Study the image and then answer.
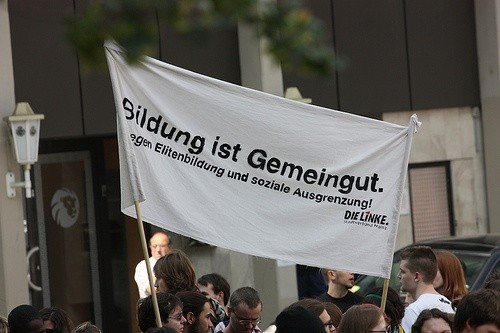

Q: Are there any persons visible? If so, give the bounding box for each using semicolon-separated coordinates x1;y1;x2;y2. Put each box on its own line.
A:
396;246;500;333
276;267;404;333
134;231;230;333
216;287;262;333
0;305;101;333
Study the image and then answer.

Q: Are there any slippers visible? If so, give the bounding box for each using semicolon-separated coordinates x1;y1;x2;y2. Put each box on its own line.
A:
167;313;183;321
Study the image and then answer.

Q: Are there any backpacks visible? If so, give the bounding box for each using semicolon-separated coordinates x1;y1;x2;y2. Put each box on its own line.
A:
204;296;230;333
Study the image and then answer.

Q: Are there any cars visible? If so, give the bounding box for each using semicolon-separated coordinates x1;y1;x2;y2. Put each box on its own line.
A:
348;233;500;298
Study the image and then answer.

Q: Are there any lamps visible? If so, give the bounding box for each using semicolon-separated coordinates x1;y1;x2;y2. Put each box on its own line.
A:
285;87;312;104
3;102;44;199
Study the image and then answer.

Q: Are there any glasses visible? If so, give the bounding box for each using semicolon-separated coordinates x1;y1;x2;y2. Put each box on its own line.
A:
322;319;334;329
230;307;262;325
